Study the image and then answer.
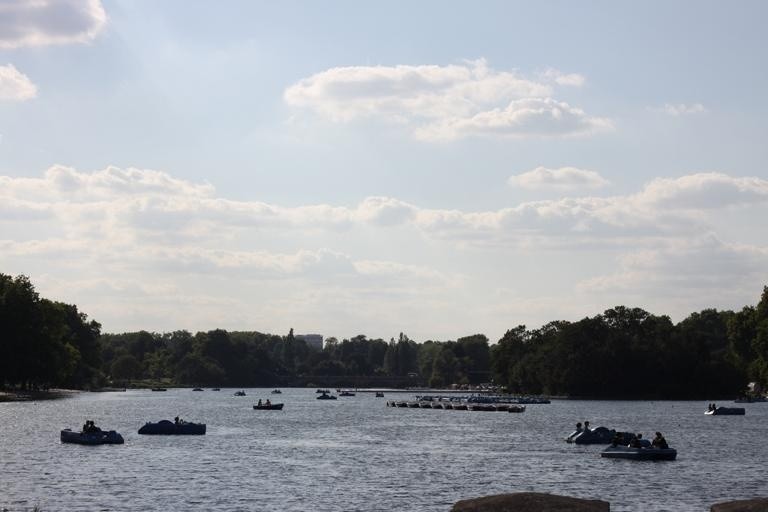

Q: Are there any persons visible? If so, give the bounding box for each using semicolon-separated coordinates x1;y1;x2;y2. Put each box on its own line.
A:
258;398;262;406
265;398;270;406
83;419;89;431
575;420;668;449
89;421;96;432
174;416;178;424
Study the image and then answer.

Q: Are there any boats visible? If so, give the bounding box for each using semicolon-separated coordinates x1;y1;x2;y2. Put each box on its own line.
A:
704;406;745;415
138;420;206;435
735;397;768;403
316;391;355;399
61;428;124;444
376;394;384;397
565;427;677;460
253;403;284;410
151;389;282;396
387;396;550;412
